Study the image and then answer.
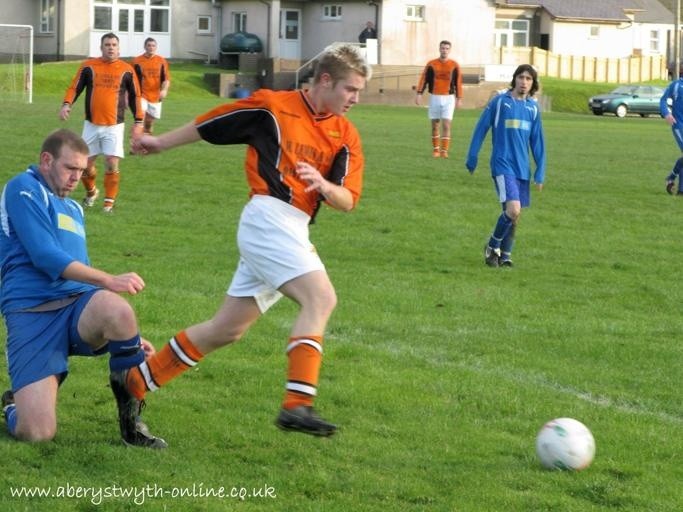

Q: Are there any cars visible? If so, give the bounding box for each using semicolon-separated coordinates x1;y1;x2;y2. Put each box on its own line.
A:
588;85;671;118
667;58;683;81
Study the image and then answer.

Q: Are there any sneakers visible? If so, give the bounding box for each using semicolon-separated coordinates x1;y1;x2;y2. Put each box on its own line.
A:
432;151;449;159
121;416;169;450
1;390;15;423
81;187;99;209
484;245;513;268
103;198;114;214
110;367;146;446
273;404;337;438
665;177;683;197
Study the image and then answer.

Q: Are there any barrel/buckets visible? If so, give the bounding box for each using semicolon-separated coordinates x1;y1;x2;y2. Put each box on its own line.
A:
235;89;250;99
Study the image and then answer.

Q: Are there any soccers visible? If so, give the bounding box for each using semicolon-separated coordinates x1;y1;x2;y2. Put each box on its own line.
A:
536;418;595;472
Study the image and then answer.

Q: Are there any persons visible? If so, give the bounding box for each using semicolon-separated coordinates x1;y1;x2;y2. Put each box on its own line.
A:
0;127;170;449
55;32;144;215
108;40;370;441
460;62;548;271
659;77;682;198
357;21;376;48
413;39;464;160
127;38;170;157
